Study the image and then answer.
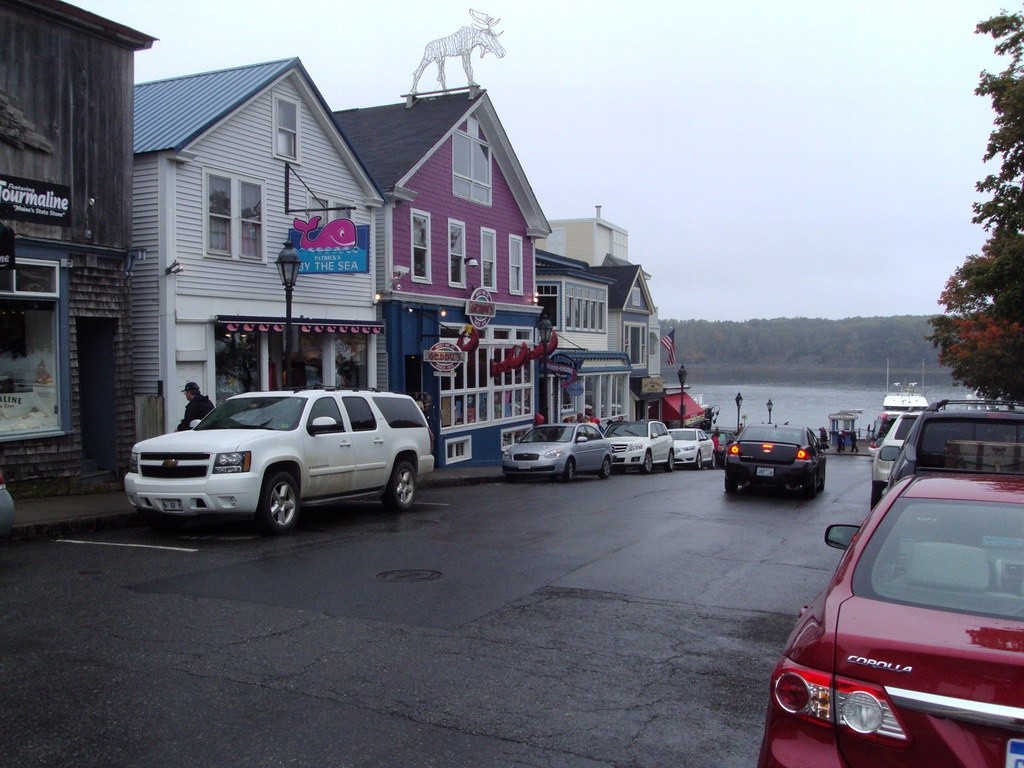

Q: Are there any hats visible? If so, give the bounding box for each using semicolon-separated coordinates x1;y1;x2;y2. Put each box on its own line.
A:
181;382;199;392
839;432;843;434
713;431;720;436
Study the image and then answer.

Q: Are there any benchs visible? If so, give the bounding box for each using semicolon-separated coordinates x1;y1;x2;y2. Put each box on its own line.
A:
896;543;1024;601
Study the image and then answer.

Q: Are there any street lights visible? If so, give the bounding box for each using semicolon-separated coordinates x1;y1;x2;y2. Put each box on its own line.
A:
275;244;303;390
678;364;687;429
735;391;742;436
766;398;774;422
537;314;553;424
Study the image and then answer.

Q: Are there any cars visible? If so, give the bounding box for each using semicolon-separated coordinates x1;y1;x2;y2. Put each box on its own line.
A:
502;423;614;480
705;430;737;470
725;425;822;496
756;470;1023;768
669;428;716;469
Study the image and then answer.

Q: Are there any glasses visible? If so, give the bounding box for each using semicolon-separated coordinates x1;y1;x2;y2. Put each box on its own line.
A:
183;393;188;395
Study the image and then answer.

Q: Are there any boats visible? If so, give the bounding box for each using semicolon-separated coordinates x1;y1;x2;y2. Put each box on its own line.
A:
884;357;928;409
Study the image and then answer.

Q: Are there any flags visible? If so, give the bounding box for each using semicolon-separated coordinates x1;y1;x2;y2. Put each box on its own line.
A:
660;327;676;369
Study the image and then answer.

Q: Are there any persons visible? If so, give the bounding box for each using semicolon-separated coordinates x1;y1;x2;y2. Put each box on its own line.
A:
607;416;624;426
174;381;215;432
710;430;720;453
739;422;744;434
818;420;876;453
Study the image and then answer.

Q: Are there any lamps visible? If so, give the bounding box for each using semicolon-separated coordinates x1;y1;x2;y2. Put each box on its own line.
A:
405;305;413;313
389;265;410;282
464;258;478;269
87;197;99;211
166;259;184;275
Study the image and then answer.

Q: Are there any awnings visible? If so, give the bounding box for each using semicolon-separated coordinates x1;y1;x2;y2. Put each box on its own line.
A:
663;392;705;425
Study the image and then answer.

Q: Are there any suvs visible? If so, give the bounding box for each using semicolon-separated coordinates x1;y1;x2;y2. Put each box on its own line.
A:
886;402;1024;520
870;410;919;511
602;419;674;473
868;411;905;458
124;389;432;534
